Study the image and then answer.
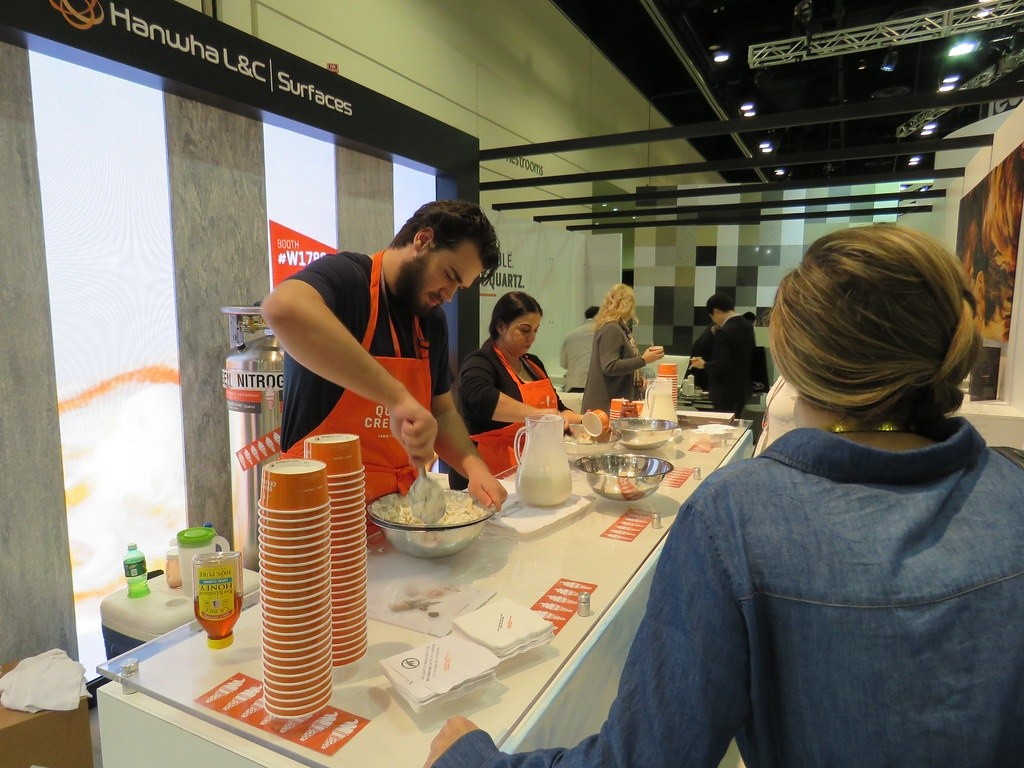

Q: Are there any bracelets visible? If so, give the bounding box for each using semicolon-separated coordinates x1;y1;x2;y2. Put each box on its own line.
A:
581;418;583;424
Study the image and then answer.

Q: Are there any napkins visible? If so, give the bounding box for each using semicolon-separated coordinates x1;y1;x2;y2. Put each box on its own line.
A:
379;599;558;714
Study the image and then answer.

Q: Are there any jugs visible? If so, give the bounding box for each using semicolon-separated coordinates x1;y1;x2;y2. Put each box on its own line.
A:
175;527;231;599
640;377;679;425
513;413;573;507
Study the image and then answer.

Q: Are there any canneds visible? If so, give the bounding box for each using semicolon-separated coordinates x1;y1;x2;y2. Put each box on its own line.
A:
165;549;183;588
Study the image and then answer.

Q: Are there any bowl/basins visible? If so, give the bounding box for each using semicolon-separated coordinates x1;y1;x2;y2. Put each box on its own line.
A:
368;487;497;559
574;453;674;502
609;417;680;451
581;409;610;438
563;423;623;462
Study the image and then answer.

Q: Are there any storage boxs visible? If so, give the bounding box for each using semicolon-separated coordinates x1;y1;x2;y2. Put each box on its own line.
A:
0;660;94;768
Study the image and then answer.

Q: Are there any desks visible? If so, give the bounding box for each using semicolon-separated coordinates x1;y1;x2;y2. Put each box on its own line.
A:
676;387;769;410
97;418;756;768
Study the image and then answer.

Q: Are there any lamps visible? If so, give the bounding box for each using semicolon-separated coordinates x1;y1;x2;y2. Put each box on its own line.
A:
879;50;900;71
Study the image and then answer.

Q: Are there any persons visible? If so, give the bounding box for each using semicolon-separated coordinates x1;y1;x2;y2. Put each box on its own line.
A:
260;199;507;538
580;284;665;417
422;225;1024;768
449;291;612;490
761;376;843;451
691;295;758;418
561;306;599;393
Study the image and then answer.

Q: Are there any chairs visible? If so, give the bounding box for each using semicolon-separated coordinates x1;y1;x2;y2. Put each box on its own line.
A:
642;354;691;385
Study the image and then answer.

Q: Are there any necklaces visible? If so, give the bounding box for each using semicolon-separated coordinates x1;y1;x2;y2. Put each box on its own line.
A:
824;419;901;434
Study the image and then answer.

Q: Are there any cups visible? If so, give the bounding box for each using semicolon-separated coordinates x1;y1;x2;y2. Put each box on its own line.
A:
657;363;678;411
702;392;709;399
649;346;663;354
609;398;644;421
690;356;701;364
256;434;369;720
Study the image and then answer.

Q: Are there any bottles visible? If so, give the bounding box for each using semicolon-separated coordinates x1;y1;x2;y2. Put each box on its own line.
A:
166;537;182;588
620;404;638;418
191;551;244;650
682;374;695;396
122;543;151;599
